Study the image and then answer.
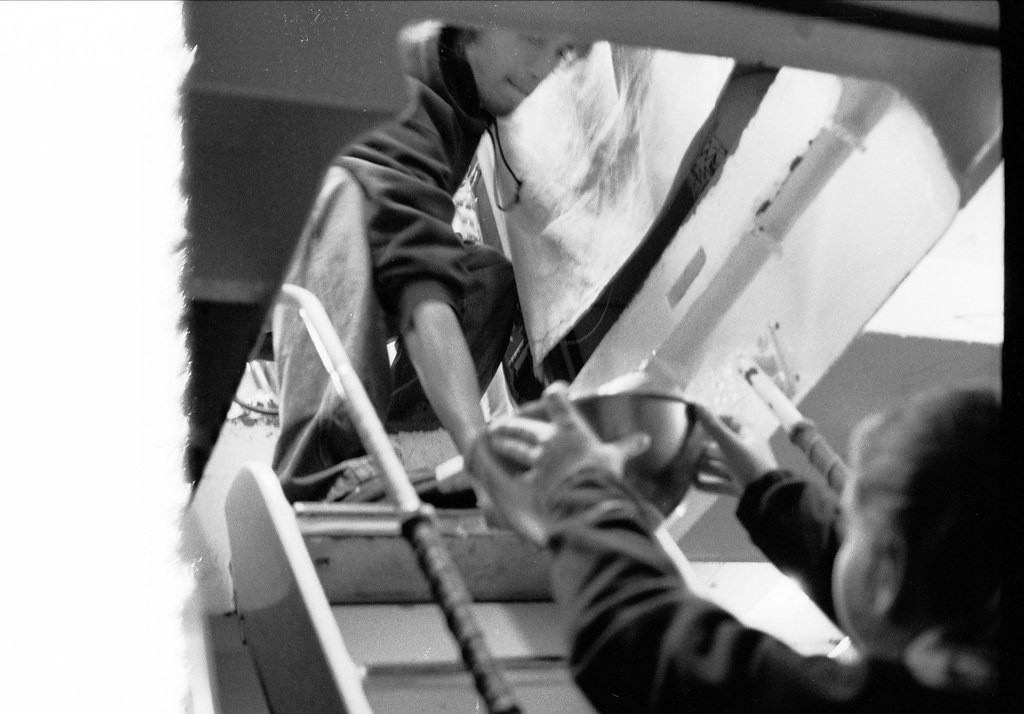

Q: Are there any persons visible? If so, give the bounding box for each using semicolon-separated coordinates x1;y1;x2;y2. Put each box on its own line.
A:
488;381;1024;714
319;20;575;531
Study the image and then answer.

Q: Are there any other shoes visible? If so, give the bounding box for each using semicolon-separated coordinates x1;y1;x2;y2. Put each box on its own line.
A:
324;455;477;510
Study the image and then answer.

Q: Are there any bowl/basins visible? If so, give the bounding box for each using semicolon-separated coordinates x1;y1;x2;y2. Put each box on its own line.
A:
491;391;710;529
